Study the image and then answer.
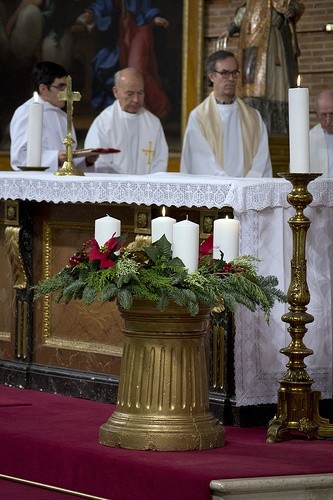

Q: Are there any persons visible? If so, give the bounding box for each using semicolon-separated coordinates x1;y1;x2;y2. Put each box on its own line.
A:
10;60;98;172
307;89;333;176
84;68;168;176
180;50;272;179
218;0;305;133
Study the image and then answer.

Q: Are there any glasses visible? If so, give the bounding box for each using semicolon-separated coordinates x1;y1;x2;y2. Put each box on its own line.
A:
48;84;67;91
213;69;240;79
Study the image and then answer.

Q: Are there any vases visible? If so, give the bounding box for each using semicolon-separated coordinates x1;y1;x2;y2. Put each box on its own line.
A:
99;295;227;451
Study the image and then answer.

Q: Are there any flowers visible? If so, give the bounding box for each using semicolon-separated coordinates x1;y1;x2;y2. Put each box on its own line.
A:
28;235;288;328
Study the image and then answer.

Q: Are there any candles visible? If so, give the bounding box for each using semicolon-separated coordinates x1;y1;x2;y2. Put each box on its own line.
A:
172;215;199;274
151;207;176;248
95;214;121;256
26;91;42;167
288;74;309;173
213;215;240;263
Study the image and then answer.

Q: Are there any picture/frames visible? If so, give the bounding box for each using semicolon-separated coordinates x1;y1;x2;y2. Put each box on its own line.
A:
0;0;204;171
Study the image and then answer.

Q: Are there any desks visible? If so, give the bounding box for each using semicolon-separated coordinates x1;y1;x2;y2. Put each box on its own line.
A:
0;170;333;428
